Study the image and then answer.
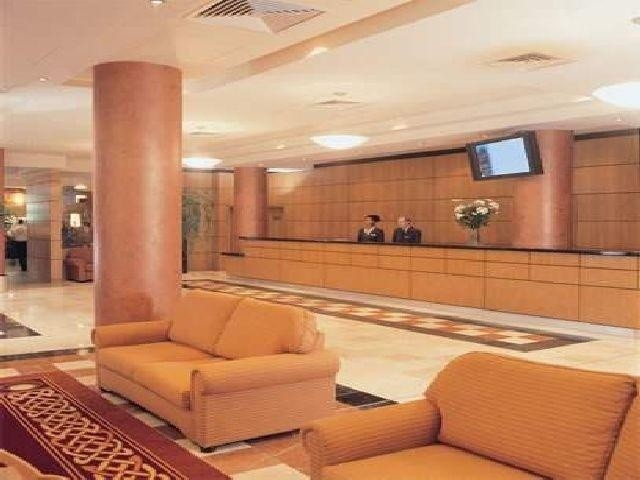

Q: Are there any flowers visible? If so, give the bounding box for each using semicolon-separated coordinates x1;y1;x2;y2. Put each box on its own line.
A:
450;197;500;231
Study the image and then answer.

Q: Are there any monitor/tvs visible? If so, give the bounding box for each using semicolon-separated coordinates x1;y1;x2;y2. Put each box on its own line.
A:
466;131;543;181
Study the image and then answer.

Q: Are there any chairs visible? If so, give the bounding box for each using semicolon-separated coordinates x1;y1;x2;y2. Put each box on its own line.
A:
65;246;93;281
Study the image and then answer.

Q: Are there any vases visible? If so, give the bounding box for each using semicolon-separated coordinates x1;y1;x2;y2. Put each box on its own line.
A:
466;228;479;244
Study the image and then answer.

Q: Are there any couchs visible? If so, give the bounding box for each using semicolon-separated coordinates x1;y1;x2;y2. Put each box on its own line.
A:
299;350;639;480
91;286;341;449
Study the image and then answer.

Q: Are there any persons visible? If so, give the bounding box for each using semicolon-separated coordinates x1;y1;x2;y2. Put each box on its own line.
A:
357;215;385;242
11;220;27;271
393;214;422;244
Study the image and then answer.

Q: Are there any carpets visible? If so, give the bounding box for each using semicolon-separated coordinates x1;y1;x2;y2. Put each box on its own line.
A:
1;369;236;480
